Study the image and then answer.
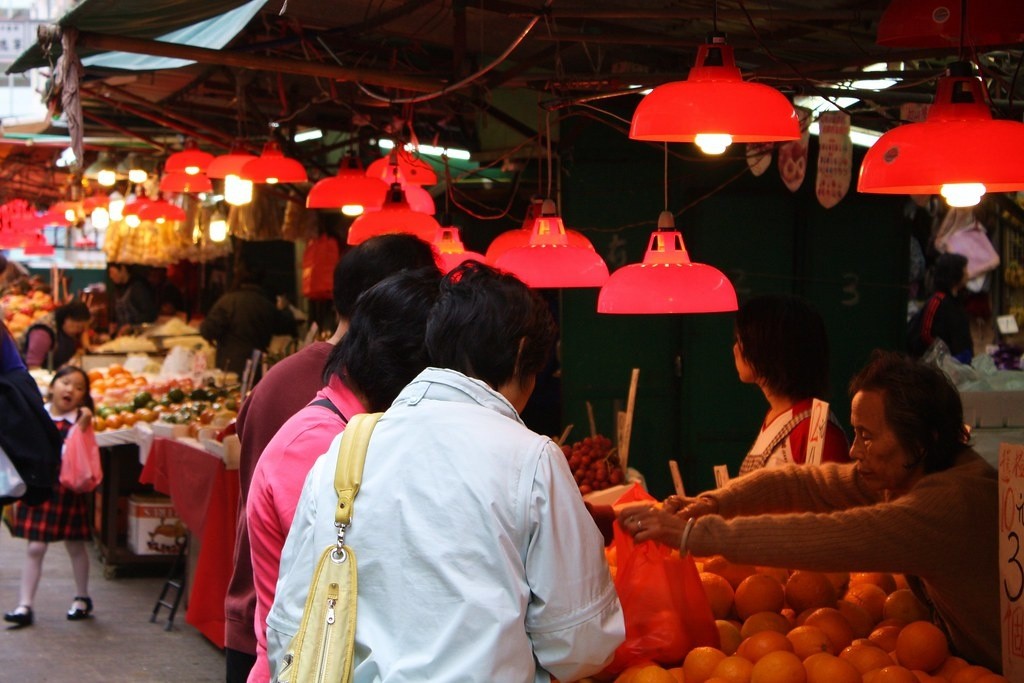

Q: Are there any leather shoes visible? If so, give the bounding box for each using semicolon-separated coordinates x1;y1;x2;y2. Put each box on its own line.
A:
4;605;32;624
68;597;92;619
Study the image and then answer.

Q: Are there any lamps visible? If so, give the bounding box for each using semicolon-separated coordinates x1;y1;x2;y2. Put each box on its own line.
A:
855;0;1024;208
594;143;740;316
628;0;802;158
0;87;611;289
874;0;1024;50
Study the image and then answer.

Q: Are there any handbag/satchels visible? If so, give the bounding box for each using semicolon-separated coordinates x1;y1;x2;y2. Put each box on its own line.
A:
942;222;1000;279
276;413;384;683
59;424;103;494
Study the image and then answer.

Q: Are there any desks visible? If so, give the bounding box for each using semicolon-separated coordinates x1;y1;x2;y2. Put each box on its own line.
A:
140;437;243;652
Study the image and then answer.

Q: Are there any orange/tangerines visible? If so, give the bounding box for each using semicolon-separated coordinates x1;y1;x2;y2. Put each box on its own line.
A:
550;546;1009;683
87;363;243;437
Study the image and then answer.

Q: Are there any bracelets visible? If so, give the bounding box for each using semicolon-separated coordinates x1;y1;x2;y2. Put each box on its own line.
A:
679;517;695;557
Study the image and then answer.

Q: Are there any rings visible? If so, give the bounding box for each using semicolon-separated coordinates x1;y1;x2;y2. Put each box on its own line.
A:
637;521;642;531
630;515;634;521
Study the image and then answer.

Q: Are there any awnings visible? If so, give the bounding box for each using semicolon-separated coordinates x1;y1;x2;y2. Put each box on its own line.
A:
383;153;537;198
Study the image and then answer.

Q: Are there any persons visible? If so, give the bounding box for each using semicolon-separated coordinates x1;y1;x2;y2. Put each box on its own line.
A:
196;234;627;683
17;303;91;372
0;254;97;626
108;264;159;323
621;293;1002;668
920;251;985;365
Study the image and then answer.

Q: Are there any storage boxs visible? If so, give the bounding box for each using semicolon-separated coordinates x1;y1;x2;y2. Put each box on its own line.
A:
960;370;1024;429
125;494;188;557
